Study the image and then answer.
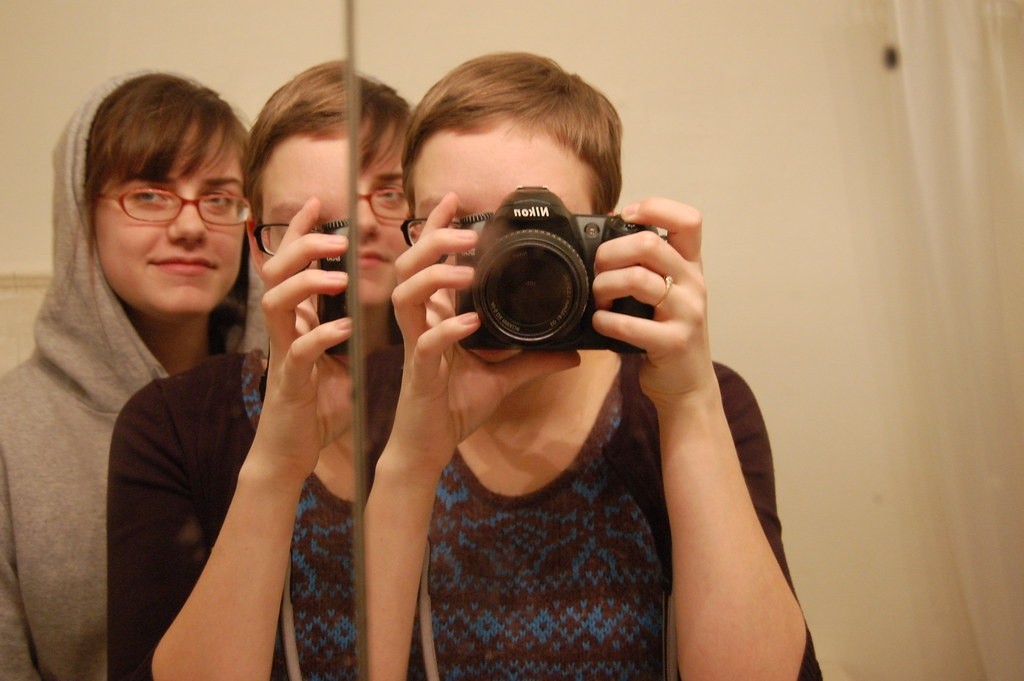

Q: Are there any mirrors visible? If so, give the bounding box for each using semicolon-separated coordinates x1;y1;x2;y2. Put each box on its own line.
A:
0;0;1024;681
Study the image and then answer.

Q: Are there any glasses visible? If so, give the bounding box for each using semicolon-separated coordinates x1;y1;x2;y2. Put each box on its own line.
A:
254;221;289;256
355;185;411;220
401;212;460;247
94;188;252;225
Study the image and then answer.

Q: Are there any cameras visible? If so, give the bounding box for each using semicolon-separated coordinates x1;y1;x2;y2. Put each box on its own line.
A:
316;251;349;357
456;185;661;353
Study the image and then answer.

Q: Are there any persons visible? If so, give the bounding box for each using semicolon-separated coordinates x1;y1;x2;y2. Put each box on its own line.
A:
1;63;412;681
361;53;829;681
